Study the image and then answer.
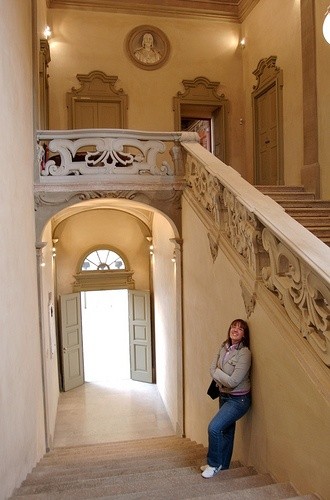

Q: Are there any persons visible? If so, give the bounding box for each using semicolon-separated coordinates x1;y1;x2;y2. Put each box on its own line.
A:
134;32;161;64
199;318;252;478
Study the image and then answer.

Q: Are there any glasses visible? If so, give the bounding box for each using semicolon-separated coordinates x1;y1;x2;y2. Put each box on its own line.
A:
231;325;243;331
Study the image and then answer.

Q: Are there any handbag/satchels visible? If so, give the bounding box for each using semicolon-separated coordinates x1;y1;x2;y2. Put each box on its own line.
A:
207;379;220;400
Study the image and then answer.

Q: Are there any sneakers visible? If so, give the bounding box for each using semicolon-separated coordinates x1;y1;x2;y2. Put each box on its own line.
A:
201;465;222;478
200;465;209;471
